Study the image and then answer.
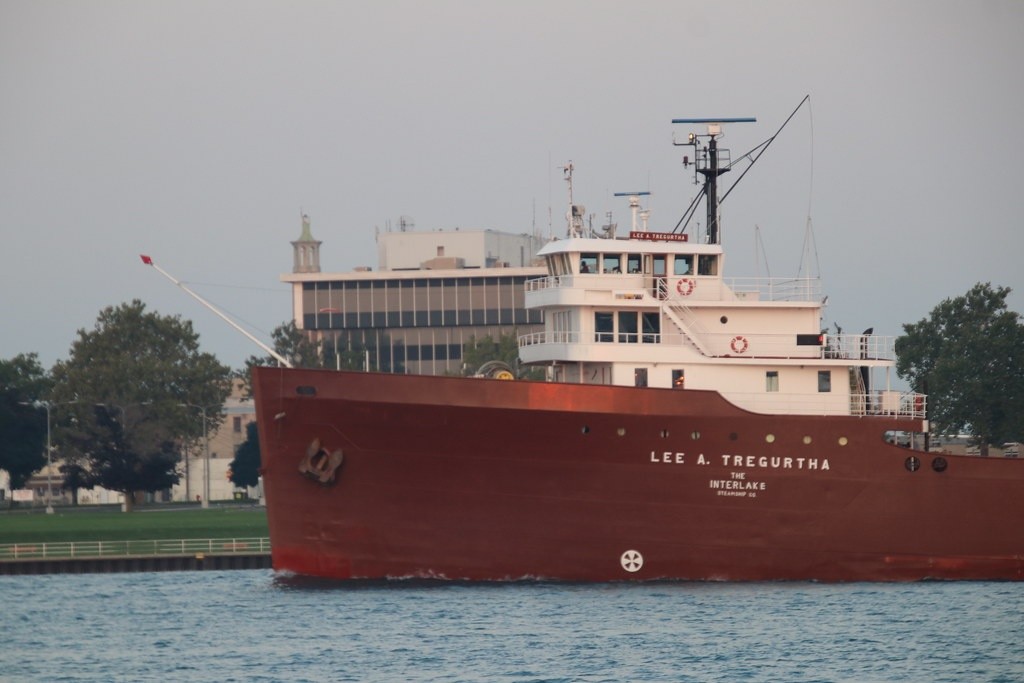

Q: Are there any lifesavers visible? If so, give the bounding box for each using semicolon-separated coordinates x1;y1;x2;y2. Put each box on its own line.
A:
730;335;749;353
676;278;694;295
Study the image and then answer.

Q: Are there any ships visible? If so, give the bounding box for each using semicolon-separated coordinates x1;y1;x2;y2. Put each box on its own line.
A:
140;94;1023;588
